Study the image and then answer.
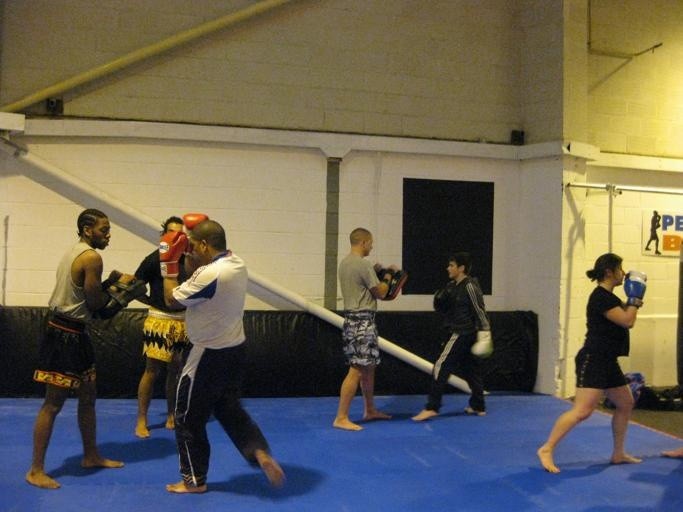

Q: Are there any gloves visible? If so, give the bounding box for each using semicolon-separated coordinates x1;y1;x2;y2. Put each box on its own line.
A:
158;213;210;278
471;330;494;360
624;270;648;306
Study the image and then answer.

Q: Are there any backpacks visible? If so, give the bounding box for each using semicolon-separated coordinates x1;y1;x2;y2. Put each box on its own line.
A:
604;372;660;411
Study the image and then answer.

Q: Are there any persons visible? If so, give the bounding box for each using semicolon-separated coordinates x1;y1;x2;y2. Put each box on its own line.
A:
129;215;189;438
22;208;147;490
535;253;647;475
411;251;494;423
158;211;286;495
332;228;409;432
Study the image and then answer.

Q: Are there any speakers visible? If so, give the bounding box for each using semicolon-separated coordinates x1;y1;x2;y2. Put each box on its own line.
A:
46;97;63;115
510;129;525;145
